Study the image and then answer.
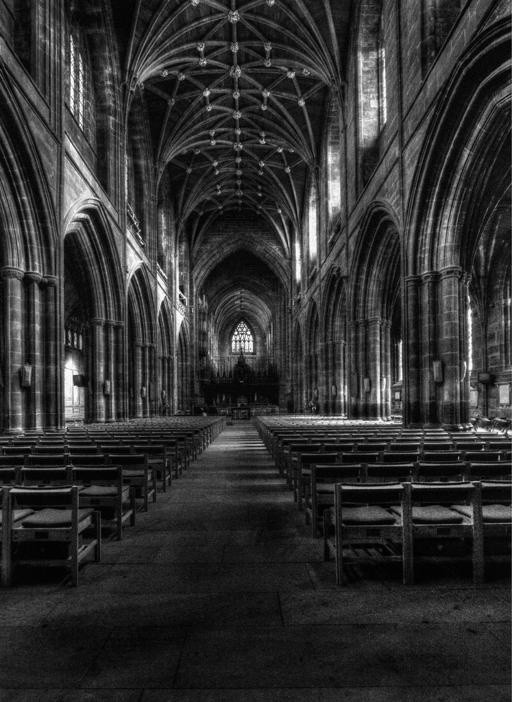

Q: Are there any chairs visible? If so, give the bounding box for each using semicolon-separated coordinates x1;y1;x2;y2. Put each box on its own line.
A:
255;415;510;589
0;415;227;590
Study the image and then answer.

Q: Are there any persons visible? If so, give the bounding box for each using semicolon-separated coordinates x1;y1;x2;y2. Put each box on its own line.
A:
287;395;293;413
